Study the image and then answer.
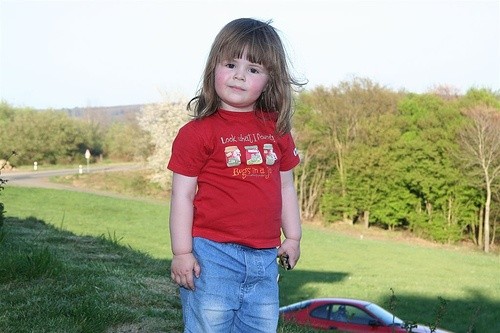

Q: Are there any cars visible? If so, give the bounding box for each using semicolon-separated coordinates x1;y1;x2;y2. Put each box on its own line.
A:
279;297;455;333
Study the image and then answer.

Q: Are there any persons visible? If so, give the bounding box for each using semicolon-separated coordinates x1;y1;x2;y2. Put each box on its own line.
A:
166;18;310;332
